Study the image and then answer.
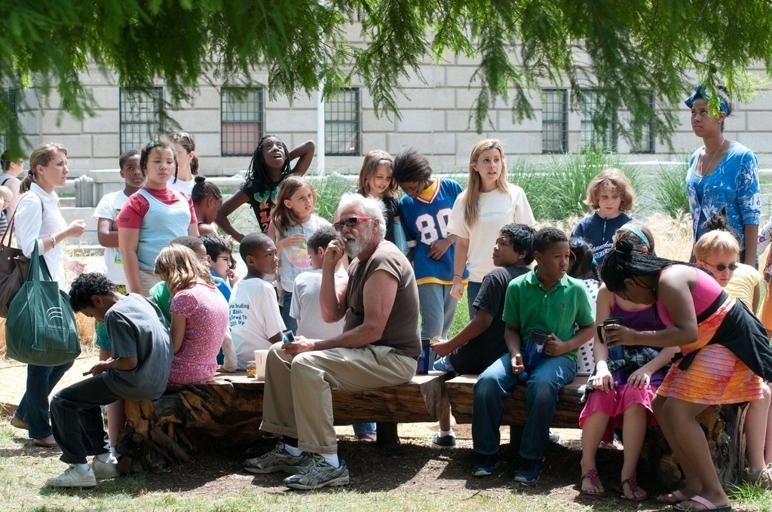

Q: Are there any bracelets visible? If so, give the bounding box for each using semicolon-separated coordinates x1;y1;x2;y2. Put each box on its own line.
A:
447;236;455;244
453;273;463;280
49;235;56;248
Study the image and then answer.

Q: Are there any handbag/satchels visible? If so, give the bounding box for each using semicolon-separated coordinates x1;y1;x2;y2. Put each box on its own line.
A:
5;280;82;367
0;244;31;318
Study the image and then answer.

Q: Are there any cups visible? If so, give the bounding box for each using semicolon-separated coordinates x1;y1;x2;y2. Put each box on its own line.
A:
415;336;437;373
521;328;548;369
596;318;627;361
254;350;269;378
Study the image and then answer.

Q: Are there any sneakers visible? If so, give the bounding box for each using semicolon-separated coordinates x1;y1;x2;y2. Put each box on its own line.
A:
514;455;545;482
472;464;495;477
433;433;455;446
243;441;311;474
91;456;120;479
32;434;57;447
46;465;97;487
11;417;29;429
357;434;376;441
282;453;350;490
743;463;772;490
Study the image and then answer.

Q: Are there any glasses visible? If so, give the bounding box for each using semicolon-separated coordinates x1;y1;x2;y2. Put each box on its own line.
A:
334;215;375;232
216;255;231;264
702;260;738;271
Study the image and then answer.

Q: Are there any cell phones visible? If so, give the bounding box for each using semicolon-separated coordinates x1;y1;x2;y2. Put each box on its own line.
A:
283;330;296;342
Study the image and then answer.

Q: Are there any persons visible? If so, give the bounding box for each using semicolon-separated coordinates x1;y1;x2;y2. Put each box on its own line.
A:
167;132;200;198
392;147;469;375
1;148;24;235
428;221;538;447
108;242;239;459
600;230;772;512
578;223;681;501
288;225;350;342
191;175;224;237
199;233;239;303
44;272;175;488
227;232;288;373
701;214;762;320
241;192;422;491
569;167;636;267
267;176;333;336
8;142;88;448
685;82;760;274
353;148;400;443
92;148;147;296
691;228;771;491
469;226;595;484
214;134;316;244
115;140;200;298
0;186;16;245
756;216;771;346
148;235;211;325
446;138;536;321
566;238;603;376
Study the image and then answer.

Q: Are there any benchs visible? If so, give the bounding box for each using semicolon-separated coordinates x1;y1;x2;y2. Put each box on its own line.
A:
444;364;751;483
115;371;446;477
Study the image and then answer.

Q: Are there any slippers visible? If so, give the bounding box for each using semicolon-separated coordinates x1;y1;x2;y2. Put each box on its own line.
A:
577;469;608;495
673;495;732;512
656;489;688;504
614;478;649;502
